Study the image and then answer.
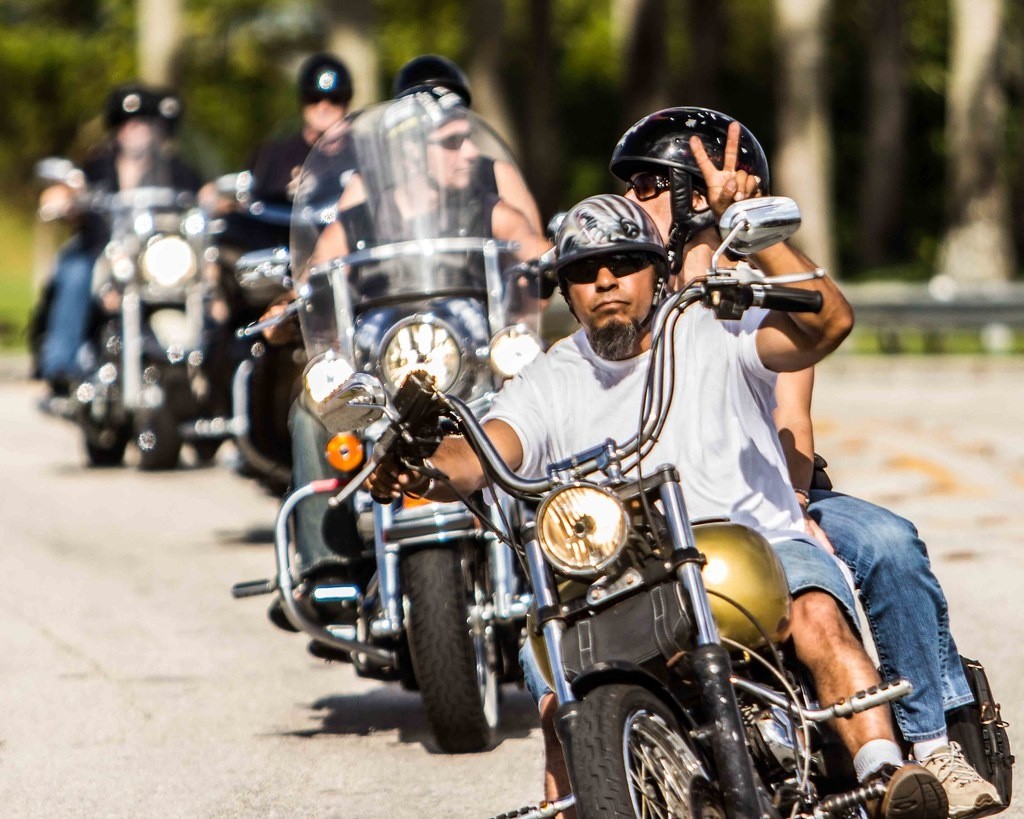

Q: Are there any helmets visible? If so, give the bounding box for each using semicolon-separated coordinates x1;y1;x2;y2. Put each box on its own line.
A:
553;194;669;306
395;54;470;107
378;84;463;146
297;54;351;101
609;106;770;199
108;88;181;128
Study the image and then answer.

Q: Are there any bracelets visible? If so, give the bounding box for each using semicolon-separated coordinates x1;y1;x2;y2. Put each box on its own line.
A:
793;488;808;499
402;458;435;500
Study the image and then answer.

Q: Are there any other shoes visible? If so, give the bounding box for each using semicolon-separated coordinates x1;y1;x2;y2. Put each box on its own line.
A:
268;573;374;632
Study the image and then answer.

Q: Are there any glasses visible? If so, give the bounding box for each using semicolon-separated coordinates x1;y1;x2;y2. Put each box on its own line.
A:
562;250;660;284
624;172;707;201
413;128;472;150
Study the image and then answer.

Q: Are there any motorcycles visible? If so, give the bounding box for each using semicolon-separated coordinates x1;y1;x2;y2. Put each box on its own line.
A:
197;108;393;490
229;82;559;757
315;198;1017;819
40;105;233;471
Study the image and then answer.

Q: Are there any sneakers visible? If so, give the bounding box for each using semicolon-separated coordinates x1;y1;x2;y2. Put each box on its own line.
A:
924;740;1004;817
862;762;950;819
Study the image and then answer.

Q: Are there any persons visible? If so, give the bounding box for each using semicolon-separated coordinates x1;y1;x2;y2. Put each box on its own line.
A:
38;84;237;411
336;52;549;331
259;88;558;630
610;106;1005;819
199;56;357;419
360;121;950;819
